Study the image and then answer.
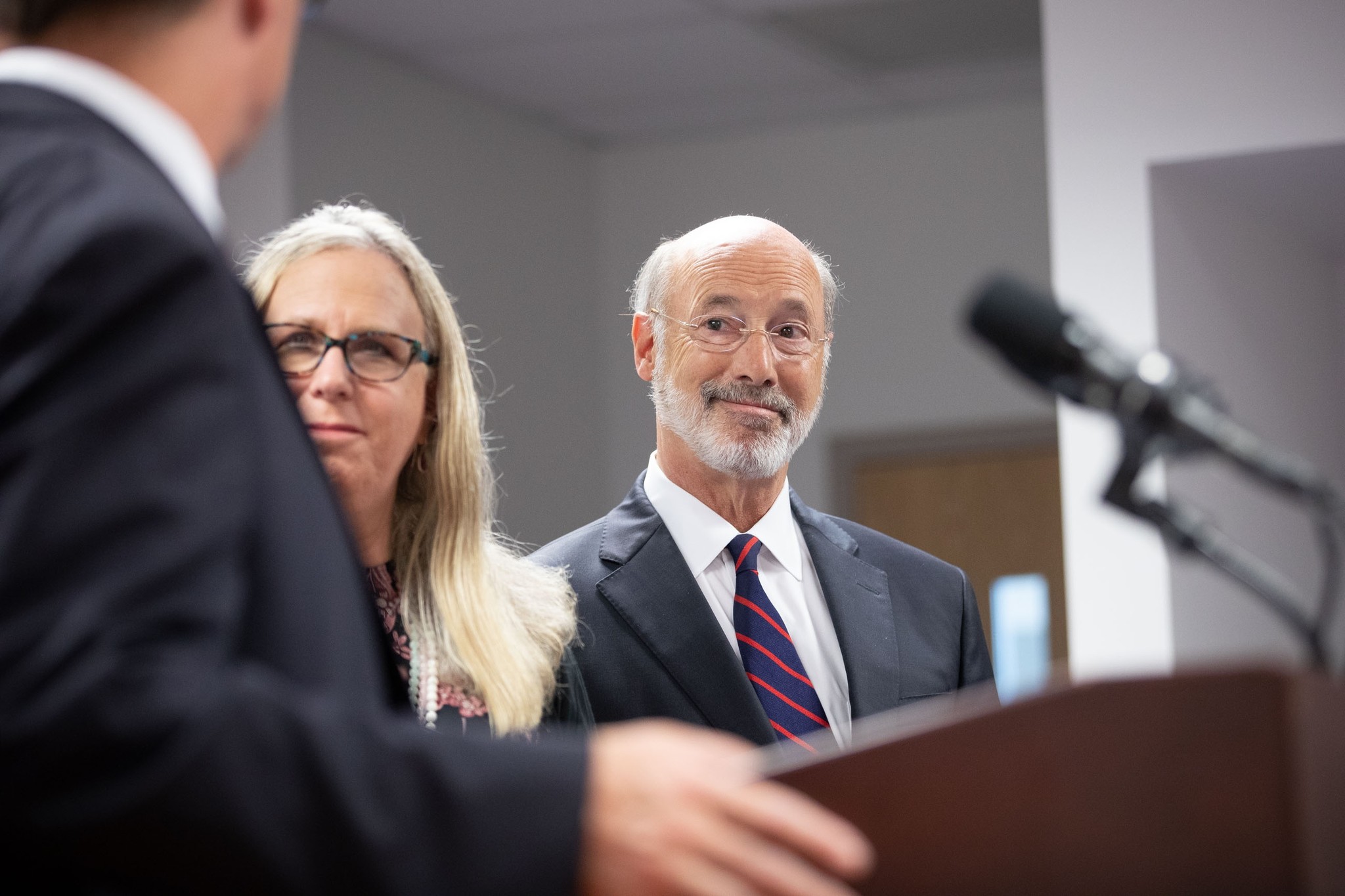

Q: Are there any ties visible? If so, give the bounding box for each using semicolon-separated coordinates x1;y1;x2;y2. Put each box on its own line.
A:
724;534;840;759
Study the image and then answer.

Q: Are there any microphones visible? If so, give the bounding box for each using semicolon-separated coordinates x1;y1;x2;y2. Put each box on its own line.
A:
966;276;1326;505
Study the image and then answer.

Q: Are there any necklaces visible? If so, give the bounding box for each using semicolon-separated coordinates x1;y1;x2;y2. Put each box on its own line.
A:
406;562;439;730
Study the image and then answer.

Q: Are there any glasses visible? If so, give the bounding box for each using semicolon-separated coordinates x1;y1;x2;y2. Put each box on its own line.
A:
647;307;830;360
264;322;439;382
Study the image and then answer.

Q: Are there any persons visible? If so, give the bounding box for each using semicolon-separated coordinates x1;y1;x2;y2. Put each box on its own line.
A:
518;214;1000;751
0;0;873;896
239;199;597;742
